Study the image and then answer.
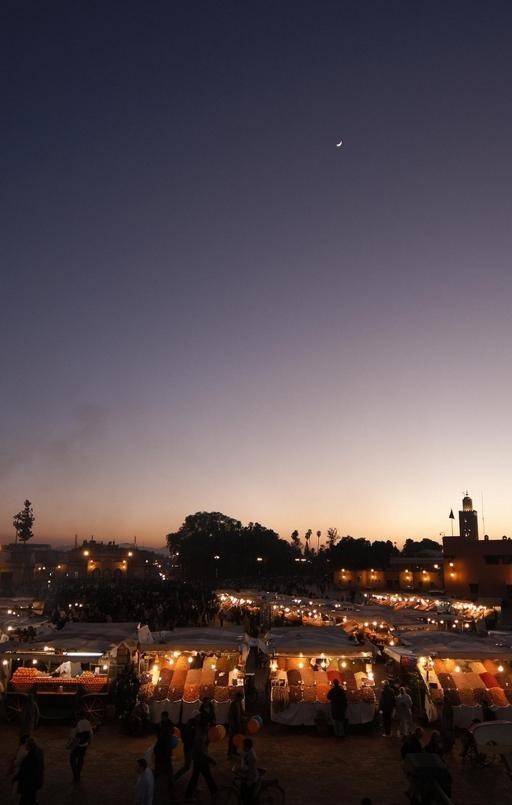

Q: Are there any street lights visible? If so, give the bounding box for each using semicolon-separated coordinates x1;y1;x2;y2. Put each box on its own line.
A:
214;555;220;579
256;557;263;592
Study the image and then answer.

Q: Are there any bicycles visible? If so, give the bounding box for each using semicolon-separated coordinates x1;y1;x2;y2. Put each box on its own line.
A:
217;777;286;805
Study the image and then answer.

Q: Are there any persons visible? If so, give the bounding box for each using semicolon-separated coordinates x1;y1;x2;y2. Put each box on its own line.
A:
109;680;286;805
327;680;485;805
12;694;93;805
